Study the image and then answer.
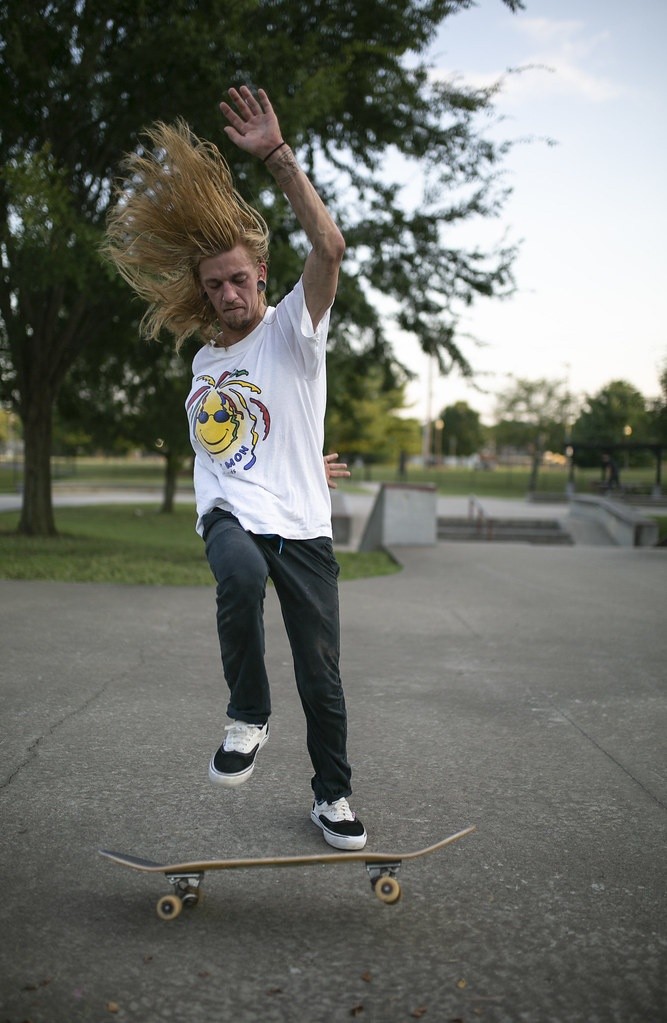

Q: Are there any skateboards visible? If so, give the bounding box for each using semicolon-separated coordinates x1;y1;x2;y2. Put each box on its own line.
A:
93;822;482;924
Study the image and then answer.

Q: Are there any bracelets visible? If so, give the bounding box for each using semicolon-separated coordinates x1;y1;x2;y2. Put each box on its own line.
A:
263;142;285;164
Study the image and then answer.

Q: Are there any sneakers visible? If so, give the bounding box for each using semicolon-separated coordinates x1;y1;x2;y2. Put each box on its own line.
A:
208;719;270;787
311;796;368;850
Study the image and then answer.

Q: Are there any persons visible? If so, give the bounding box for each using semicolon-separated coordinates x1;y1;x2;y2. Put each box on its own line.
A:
600;451;609;480
607;458;620;487
104;84;368;849
